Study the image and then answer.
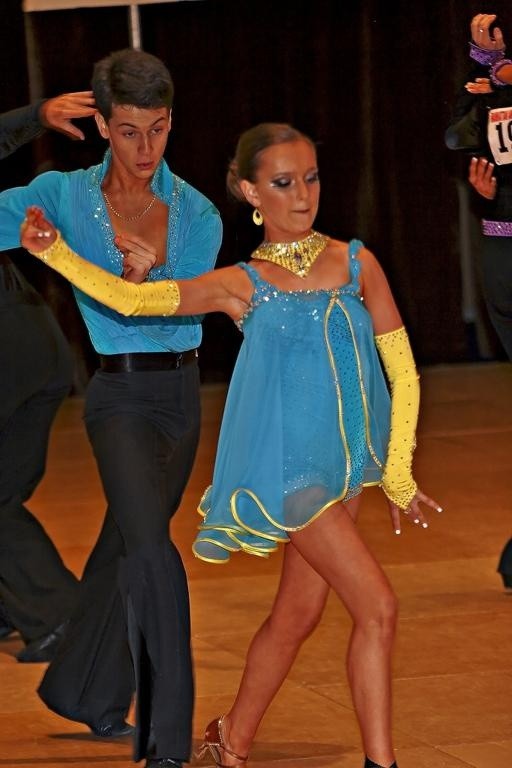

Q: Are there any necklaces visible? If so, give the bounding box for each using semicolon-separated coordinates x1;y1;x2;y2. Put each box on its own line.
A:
251;230;334;283
101;188;159;223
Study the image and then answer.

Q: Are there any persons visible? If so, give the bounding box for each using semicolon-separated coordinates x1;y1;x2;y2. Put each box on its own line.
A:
0;89;99;667
18;121;446;768
0;47;221;768
443;14;510;590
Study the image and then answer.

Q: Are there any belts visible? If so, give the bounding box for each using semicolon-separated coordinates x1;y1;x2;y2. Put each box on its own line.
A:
99;349;196;373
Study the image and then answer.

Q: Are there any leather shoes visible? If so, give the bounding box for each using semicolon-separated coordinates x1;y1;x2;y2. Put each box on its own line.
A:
145;759;183;768
87;722;135;737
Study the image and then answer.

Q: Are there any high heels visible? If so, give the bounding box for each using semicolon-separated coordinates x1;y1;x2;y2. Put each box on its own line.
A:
196;713;248;768
